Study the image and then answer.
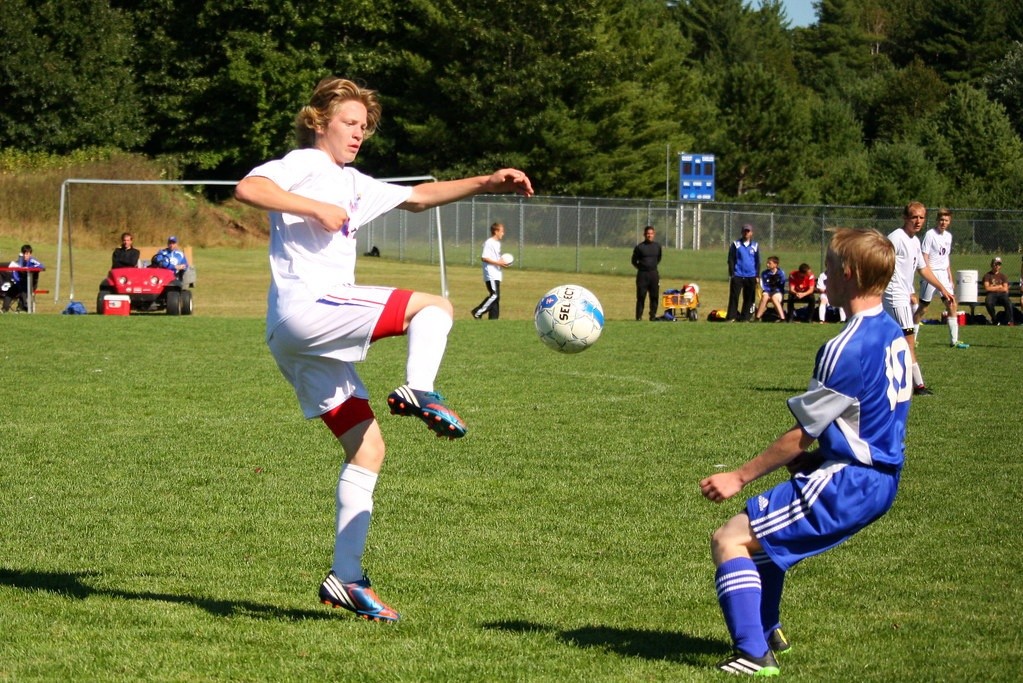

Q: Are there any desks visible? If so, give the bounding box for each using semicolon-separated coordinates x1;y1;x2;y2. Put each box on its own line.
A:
0;266;46;315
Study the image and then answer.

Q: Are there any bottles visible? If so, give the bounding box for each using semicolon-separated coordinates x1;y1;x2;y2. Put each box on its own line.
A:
18;252;22;268
143;260;147;269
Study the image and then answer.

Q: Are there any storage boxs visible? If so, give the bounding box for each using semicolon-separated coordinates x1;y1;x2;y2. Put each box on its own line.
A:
662;293;698;309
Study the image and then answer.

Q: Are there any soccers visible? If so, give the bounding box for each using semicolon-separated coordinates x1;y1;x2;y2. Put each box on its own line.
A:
533;285;606;354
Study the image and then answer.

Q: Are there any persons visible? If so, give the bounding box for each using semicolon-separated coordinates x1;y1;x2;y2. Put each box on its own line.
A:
753;256;786;323
471;222;509;320
982;256;1013;326
911;287;923;325
914;208;969;348
1019;264;1023;311
816;272;846;324
157;236;188;274
725;224;760;323
882;201;955;395
112;232;140;268
235;78;533;624
0;245;41;313
631;225;662;320
785;263;816;323
700;228;912;677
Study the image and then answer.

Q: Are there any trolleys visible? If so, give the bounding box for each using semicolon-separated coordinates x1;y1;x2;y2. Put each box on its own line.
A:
662;292;699;322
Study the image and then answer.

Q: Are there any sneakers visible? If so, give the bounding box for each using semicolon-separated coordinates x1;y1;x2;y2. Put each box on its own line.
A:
387;385;467;440
711;648;778;677
766;629;790;654
950;340;969;348
317;570;397;624
913;383;933;394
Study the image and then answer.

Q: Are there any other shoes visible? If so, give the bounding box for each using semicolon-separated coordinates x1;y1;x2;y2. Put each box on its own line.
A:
1008;321;1014;326
649;317;661;320
470;310;481;318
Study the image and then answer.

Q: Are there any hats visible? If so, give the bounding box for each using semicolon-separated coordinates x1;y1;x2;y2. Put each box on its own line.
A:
168;236;176;242
742;224;752;231
991;257;1002;264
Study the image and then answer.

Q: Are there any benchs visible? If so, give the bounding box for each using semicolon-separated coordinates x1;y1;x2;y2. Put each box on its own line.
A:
958;279;1023;319
766;277;820;314
